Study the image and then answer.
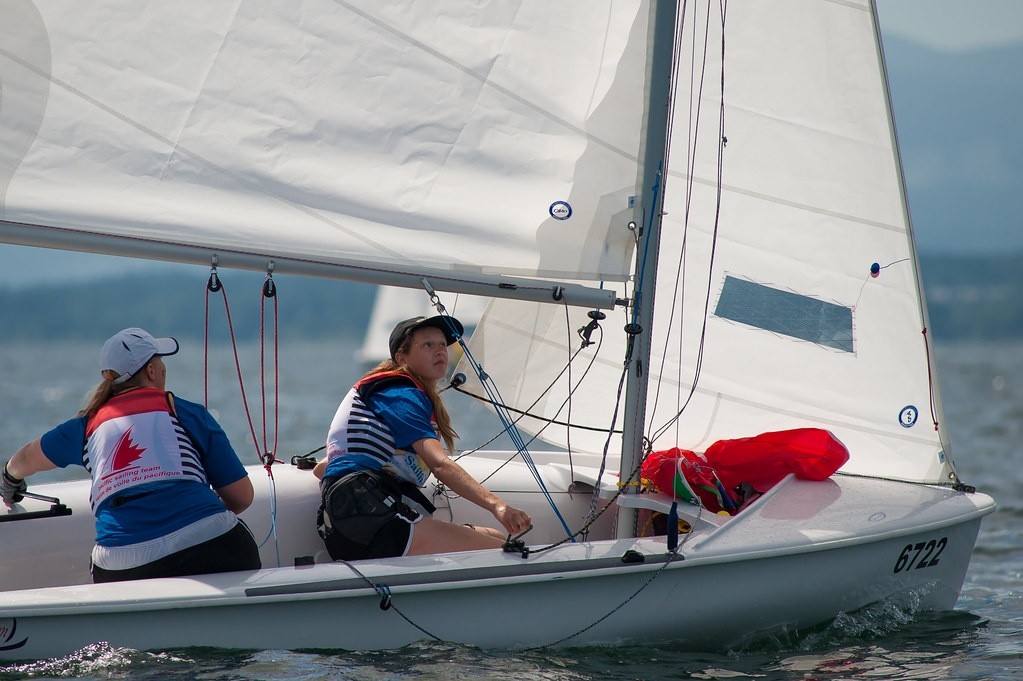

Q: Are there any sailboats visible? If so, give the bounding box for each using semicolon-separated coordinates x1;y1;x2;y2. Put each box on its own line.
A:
0;0;996;654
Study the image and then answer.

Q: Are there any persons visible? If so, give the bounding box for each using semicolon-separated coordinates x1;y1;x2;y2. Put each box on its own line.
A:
312;316;531;563
0;327;261;583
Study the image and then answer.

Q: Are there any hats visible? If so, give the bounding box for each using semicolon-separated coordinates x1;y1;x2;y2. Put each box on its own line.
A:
389;314;464;361
99;328;180;385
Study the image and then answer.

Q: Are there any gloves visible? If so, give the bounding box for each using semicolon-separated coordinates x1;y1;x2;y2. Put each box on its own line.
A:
0;461;28;505
